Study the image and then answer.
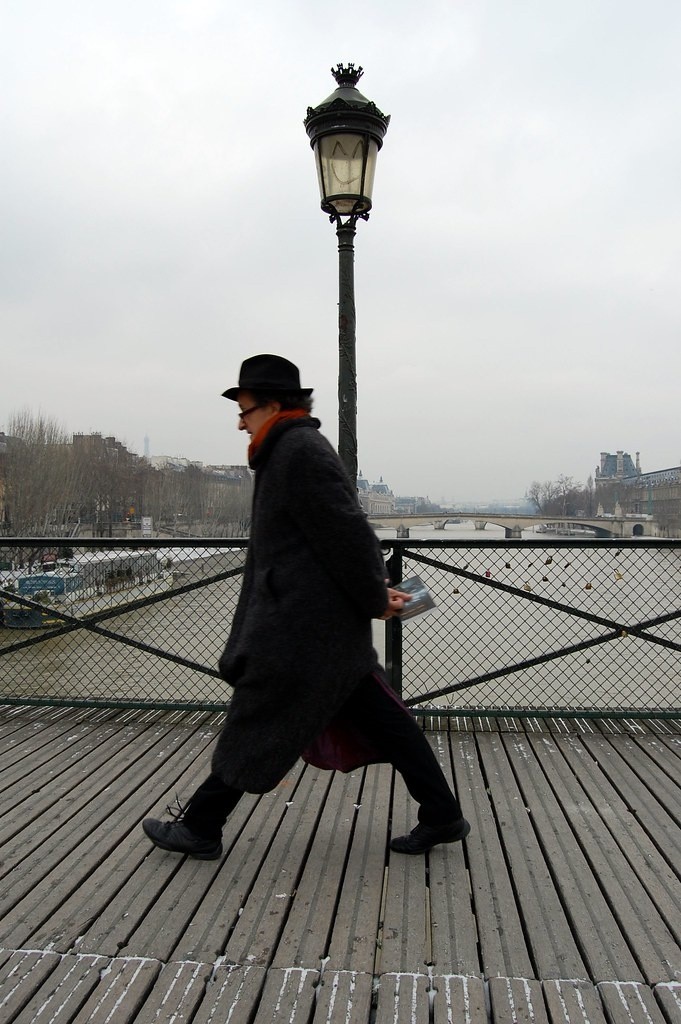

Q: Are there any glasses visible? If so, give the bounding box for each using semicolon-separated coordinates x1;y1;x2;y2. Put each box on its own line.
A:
238;399;272;419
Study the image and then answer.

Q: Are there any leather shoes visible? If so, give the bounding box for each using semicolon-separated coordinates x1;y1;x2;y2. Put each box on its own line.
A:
140;817;223;860
388;817;472;854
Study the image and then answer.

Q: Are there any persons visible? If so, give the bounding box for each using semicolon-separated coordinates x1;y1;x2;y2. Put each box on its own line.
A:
141;355;471;864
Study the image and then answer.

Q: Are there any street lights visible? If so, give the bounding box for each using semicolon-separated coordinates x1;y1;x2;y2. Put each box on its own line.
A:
305;59;395;506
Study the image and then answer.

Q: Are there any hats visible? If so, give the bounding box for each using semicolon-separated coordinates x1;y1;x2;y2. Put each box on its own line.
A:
222;354;313;402
